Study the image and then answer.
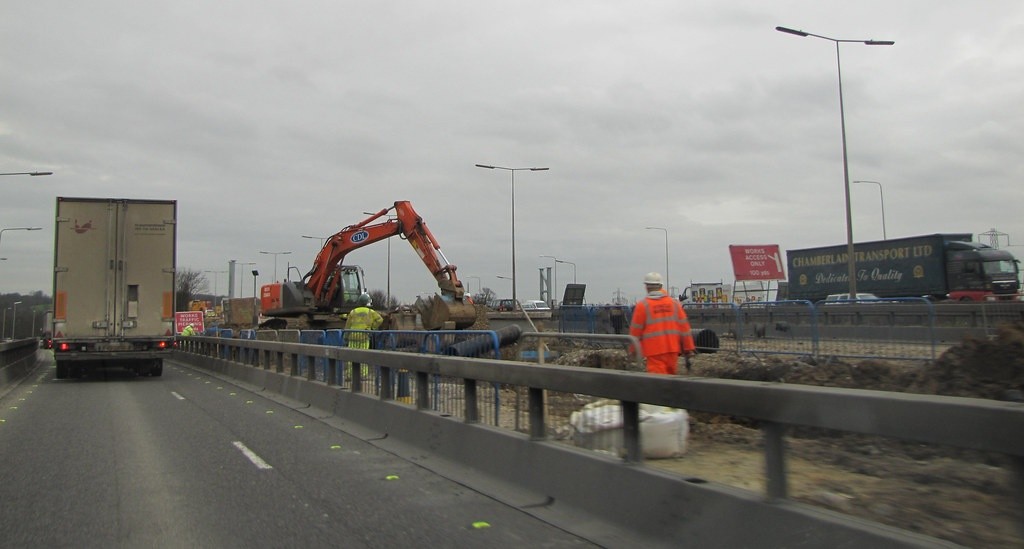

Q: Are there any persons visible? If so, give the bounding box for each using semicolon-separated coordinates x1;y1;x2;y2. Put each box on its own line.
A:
343;293;383;382
629;272;695;375
465;293;473;303
181;322;195;345
343;272;358;302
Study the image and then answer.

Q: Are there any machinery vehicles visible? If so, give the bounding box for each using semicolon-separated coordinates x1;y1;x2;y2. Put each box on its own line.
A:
256;198;477;365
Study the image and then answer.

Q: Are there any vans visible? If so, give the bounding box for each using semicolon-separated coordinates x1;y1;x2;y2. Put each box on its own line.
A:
825;292;881;304
517;300;553;311
490;298;515;311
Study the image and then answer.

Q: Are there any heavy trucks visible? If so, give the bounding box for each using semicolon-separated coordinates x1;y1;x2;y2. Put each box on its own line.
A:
786;232;1021;306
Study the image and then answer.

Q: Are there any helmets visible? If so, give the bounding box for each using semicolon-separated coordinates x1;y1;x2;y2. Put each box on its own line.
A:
358;293;371;307
190;323;194;328
644;272;663;285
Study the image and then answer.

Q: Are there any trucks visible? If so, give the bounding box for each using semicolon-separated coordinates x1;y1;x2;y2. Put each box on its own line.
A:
50;195;180;373
41;309;54;349
190;298;228;312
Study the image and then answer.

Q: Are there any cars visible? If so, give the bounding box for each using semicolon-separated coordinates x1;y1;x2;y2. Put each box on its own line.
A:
205;309;217;318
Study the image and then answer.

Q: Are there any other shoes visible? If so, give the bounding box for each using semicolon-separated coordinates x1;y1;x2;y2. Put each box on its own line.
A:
361;377;367;381
345;377;351;382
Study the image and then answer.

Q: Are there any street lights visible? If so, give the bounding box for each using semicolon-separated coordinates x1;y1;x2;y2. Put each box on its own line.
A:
644;225;671;296
775;25;897;303
302;235;326;250
2;308;13;342
539;254;562;311
466;276;482;293
233;261;257;297
362;211;397;317
203;270;228;308
555;259;576;284
473;163;549;313
259;251;292;282
12;301;23;342
